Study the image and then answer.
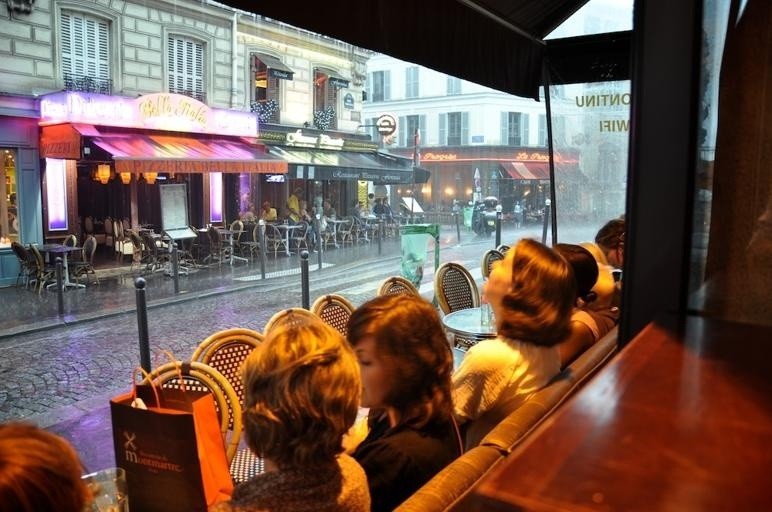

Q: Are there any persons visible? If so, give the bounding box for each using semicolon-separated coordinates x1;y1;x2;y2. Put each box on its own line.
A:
450;239;577;454
554;219;626;370
212;321;371;512
514;201;523;228
0;422;92;512
345;293;464;512
241;188;394;252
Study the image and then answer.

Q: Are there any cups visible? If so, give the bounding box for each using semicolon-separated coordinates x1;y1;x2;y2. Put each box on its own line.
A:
480;294;493;326
84;467;131;512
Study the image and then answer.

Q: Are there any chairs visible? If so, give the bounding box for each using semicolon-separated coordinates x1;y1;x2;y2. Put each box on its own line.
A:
9;200;432;294
145;245;622;493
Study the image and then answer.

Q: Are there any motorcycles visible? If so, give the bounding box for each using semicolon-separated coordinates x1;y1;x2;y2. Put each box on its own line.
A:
471;196;499;238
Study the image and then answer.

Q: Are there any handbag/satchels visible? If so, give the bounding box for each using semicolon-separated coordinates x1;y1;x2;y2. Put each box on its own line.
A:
109;349;236;511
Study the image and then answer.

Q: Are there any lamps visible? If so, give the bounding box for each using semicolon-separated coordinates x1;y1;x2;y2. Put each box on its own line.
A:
92;160;158;187
